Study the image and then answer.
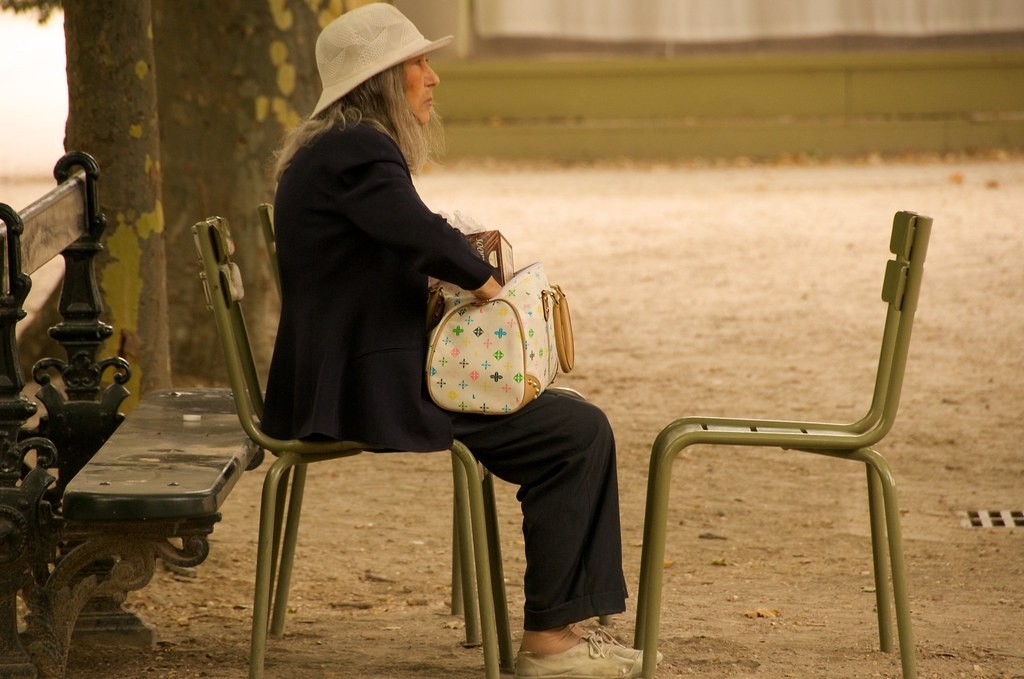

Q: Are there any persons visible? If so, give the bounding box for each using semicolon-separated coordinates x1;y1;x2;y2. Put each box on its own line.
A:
258;2;663;679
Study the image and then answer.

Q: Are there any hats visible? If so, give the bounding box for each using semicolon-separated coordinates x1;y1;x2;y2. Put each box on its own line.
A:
307;1;455;122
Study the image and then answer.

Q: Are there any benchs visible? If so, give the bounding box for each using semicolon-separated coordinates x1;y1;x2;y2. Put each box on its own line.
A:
0;150;264;678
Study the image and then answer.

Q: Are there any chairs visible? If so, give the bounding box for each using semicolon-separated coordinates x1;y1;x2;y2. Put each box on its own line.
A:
183;213;515;679
628;208;937;679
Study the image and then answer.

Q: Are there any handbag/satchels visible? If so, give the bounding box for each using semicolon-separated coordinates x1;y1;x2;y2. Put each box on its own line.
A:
424;262;575;415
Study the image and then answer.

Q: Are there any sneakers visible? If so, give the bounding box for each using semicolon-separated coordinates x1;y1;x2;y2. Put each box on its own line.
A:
580;630;664;666
513;641;657;678
